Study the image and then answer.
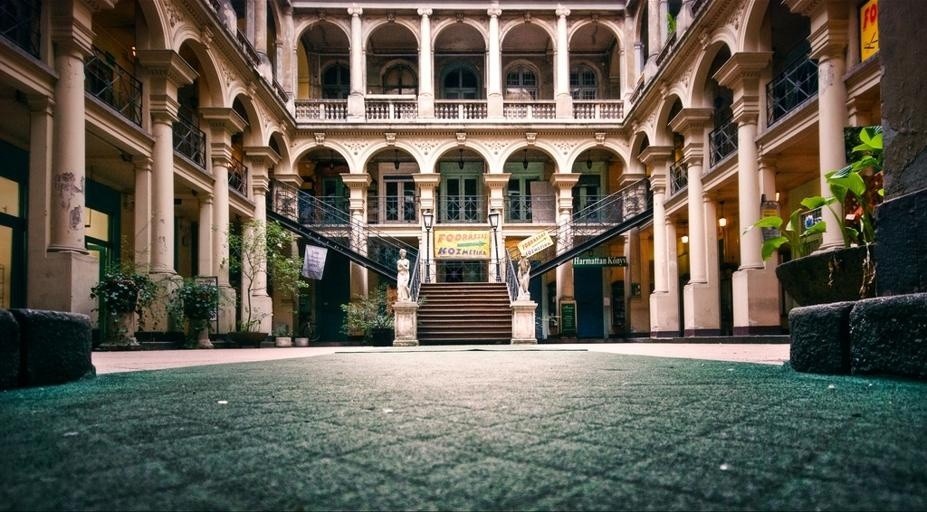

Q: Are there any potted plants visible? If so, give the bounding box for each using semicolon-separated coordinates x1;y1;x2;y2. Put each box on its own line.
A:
737;124;883;305
89;218;313;351
335;281;429;347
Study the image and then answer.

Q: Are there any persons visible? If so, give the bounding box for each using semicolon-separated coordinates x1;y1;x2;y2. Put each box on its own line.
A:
516;254;534;301
395;247;410;303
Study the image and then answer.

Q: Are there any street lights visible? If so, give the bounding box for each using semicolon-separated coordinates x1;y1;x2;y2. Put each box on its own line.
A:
422;209;434;284
487;208;503;283
